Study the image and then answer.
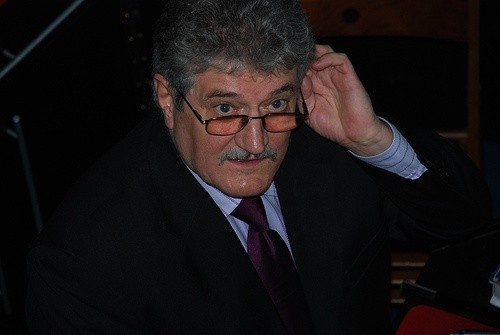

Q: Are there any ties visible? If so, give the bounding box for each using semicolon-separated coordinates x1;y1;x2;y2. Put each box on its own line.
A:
231;196;314;335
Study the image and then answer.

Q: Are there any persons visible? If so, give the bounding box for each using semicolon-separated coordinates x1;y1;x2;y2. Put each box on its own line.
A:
81;0;445;335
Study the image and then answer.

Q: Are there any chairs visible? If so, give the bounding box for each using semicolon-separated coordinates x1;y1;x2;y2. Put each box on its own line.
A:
296;0;481;298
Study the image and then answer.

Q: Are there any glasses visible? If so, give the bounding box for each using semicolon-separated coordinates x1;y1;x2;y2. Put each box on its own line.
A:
173;84;310;136
